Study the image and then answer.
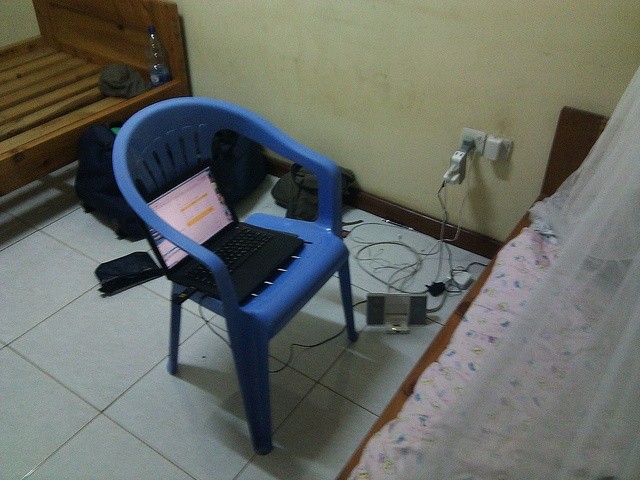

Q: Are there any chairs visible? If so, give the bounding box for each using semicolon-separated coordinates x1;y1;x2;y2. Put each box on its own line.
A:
111;96;359;454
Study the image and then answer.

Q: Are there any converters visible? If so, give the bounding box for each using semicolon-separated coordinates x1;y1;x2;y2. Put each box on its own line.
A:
483;134;503;163
440;151;470;187
448;268;473;290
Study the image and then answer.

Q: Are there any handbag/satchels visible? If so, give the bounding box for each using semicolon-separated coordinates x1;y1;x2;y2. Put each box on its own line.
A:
73;122;270;243
271;158;357;223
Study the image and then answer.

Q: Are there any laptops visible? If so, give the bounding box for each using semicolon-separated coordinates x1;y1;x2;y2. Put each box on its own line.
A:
139;159;304;303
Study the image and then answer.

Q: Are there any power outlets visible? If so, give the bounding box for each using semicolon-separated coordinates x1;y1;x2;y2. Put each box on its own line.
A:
482;133;515;162
459;126;488;155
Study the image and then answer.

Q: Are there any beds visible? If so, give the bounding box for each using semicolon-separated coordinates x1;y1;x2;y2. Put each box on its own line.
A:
335;104;640;479
0;0;194;192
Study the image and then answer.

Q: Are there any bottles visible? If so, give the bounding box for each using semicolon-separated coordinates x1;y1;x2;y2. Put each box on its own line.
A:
146;26;172;88
